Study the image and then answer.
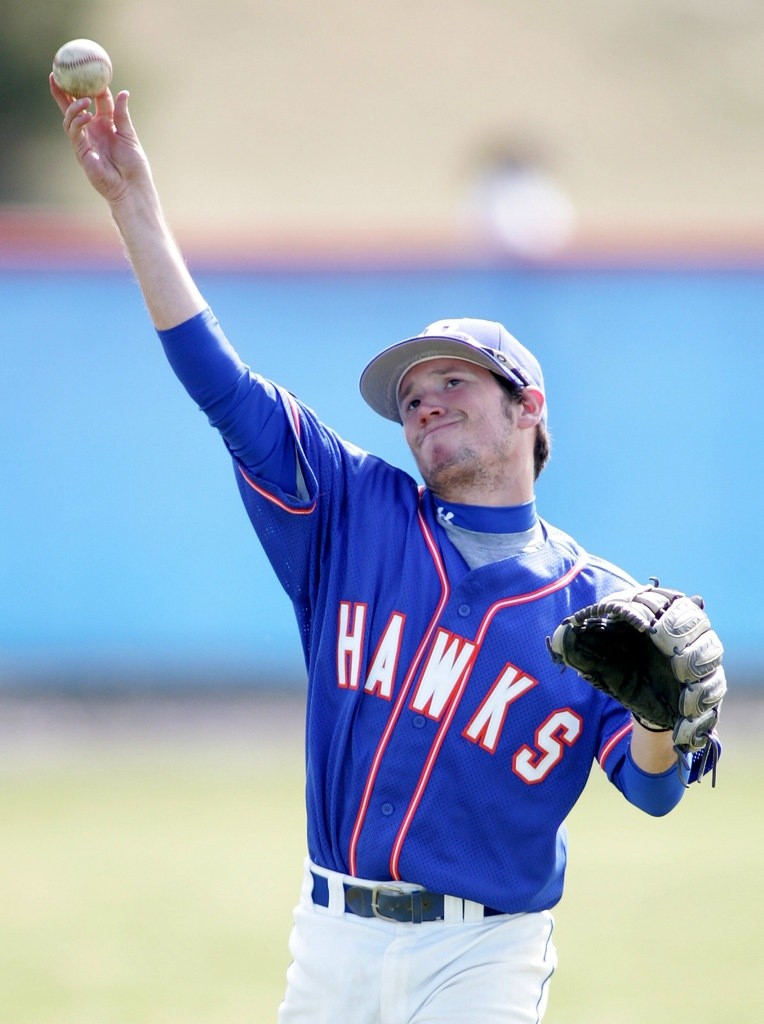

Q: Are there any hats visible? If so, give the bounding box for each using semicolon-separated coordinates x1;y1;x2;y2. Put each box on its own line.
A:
361;318;548;426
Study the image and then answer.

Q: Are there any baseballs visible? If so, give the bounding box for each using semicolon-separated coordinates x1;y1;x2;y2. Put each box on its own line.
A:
52;36;115;101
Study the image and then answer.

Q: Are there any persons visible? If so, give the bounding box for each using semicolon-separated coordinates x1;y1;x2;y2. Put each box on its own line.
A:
49;72;727;1024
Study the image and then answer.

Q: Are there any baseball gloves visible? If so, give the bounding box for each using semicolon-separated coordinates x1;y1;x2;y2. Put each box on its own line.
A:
543;576;731;756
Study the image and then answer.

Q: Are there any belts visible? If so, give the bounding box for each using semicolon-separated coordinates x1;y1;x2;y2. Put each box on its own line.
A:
308;871;507;922
